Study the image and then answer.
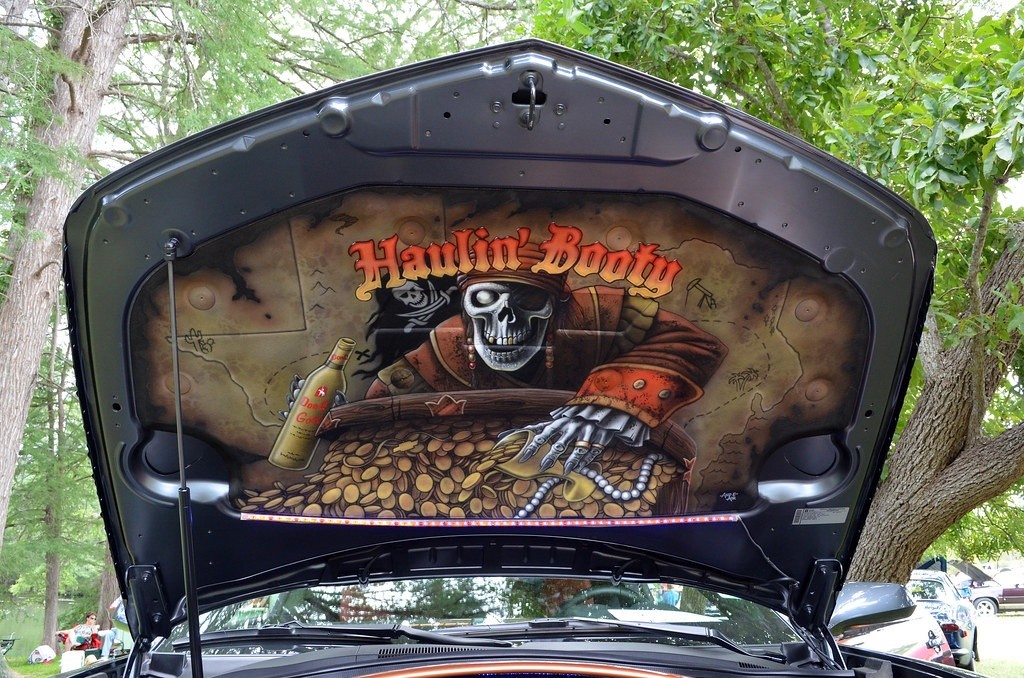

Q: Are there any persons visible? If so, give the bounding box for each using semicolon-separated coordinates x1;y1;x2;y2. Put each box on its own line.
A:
64;612;98;651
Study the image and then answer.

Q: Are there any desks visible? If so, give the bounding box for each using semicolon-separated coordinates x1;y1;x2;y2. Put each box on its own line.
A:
0;638;22;656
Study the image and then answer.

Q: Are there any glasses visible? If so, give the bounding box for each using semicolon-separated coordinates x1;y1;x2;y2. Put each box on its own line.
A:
89;616;96;620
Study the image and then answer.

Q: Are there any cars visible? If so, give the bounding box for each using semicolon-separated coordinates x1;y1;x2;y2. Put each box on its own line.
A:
905;567;982;671
944;559;1024;619
56;31;940;678
838;595;962;671
